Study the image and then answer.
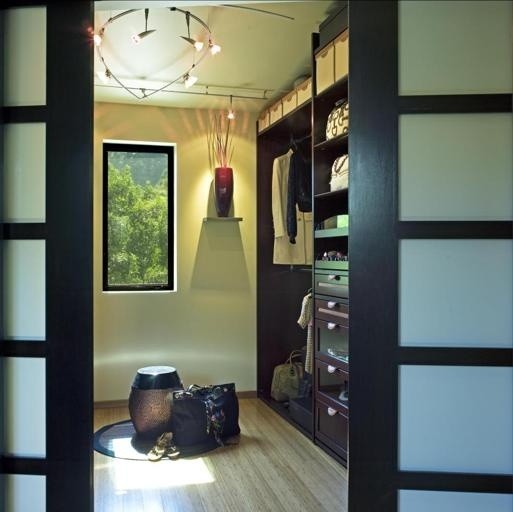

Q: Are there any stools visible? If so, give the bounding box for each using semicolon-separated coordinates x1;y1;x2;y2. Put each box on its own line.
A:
128;366;183;441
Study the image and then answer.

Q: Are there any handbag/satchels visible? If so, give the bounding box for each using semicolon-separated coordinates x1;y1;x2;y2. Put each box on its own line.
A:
271;363;306;402
325;99;349;141
170;383;241;445
330;154;350;191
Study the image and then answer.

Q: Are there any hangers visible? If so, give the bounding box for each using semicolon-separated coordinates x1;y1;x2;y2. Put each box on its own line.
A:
308;288;312;293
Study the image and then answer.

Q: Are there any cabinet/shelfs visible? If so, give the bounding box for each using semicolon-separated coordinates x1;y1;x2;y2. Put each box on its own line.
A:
253;78;312;440
310;33;349;470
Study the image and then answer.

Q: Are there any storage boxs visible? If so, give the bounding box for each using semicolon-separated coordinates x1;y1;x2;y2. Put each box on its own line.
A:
318;1;349;47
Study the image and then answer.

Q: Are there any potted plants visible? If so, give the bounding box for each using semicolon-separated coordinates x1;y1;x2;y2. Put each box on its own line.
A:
206;110;233;218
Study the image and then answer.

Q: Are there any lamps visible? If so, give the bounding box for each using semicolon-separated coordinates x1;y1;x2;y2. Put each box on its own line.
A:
93;7;221;98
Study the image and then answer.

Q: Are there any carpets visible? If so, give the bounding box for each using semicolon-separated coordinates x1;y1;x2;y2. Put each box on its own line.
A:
94;419;221;460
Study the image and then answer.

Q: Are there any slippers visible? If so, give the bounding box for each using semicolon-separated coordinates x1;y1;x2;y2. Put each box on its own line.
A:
148;432;180;461
